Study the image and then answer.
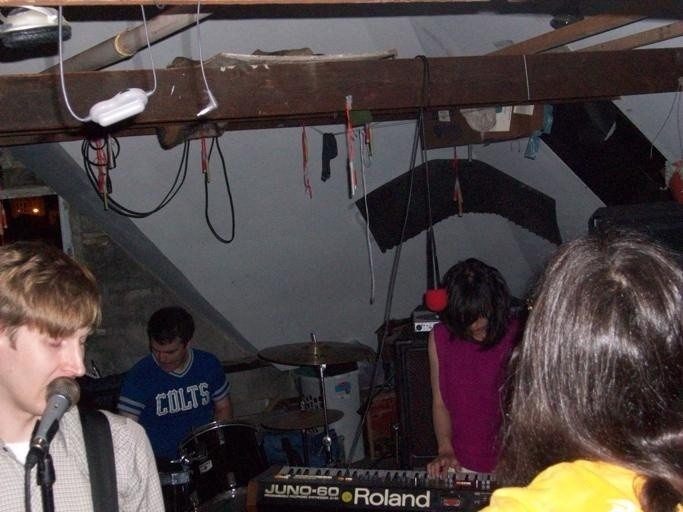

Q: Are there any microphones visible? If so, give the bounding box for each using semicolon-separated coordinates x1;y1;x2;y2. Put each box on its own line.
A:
24;376;80;465
425;227;448;311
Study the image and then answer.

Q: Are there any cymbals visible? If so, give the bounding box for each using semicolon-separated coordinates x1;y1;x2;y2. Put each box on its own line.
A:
257;342;373;366
260;408;344;430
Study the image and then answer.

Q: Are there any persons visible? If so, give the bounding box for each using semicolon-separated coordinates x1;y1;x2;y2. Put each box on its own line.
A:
115;304;233;510
471;222;683;512
0;238;164;511
426;257;528;481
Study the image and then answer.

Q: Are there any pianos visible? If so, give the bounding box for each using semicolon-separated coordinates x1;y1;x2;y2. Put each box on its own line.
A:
256;463;499;512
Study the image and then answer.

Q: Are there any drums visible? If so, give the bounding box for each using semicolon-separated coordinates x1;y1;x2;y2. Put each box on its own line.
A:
177;419;269;511
